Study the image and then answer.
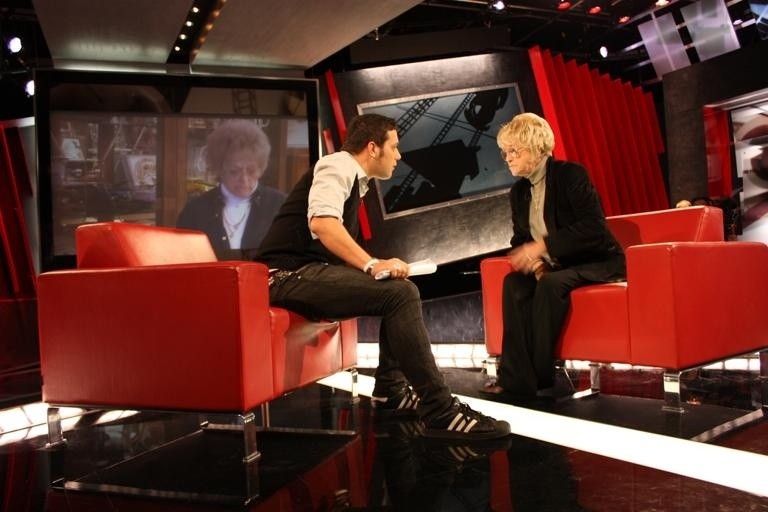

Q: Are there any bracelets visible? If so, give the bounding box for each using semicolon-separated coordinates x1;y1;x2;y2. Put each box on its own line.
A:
364;257;380;276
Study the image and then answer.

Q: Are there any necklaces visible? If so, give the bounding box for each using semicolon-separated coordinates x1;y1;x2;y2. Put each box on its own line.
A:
527;183;543;211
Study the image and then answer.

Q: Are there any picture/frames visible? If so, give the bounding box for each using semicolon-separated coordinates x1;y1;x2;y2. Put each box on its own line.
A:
354;81;527;223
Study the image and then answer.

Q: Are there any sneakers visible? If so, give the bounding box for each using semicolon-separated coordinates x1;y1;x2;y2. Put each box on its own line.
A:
370;384;422;418
418;395;511;440
476;383;508;396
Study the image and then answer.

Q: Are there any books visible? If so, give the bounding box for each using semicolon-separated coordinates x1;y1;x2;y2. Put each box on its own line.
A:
373;256;439;282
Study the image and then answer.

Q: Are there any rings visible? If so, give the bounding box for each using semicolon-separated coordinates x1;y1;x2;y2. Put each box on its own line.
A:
526;254;534;262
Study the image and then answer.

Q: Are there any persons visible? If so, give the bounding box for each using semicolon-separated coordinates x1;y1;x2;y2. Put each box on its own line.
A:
475;111;627;404
177;118;291;261
243;113;512;444
135;158;159;188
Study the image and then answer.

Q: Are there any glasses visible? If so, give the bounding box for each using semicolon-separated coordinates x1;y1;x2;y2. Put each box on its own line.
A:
221;166;264;181
499;146;525;162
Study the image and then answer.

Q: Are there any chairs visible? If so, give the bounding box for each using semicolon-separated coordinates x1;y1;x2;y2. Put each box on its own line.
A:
33;219;362;463
489;380;768;511
44;415;374;511
478;204;767;416
